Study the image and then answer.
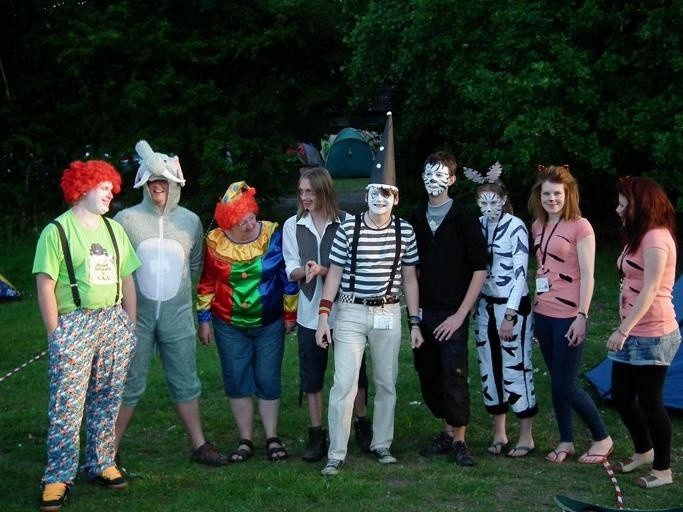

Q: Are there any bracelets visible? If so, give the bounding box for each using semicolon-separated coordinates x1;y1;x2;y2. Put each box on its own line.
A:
615;327;627;337
408;316;421;329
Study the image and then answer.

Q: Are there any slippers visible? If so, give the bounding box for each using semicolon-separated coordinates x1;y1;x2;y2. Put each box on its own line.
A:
633;472;674;489
612;457;652;473
506;443;535;459
487;440;510;456
544;447;576;463
578;443;614;466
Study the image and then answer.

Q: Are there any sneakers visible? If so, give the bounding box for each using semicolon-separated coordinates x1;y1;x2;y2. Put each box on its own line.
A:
302;426;328;462
321;457;346;477
355;421;373;456
41;481;71;508
92;466;129;491
371;447;397;464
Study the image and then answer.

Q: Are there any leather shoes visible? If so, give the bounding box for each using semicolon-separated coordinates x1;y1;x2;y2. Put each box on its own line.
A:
448;441;479;466
419;433;454;458
190;444;229;467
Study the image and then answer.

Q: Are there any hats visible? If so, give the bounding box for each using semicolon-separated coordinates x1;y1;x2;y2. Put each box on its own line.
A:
364;110;400;193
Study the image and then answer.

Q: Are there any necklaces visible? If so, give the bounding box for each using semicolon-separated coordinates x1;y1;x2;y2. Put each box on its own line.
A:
367;213;391;230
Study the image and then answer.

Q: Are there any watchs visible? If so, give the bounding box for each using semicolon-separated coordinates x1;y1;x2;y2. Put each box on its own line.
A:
505;313;517;321
578;311;588;319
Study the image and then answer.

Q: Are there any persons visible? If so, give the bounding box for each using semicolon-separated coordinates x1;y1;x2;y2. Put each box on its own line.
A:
197;181;299;462
405;151;490;467
112;139;229;466
607;174;682;489
314;110;424;475
463;161;537;457
531;165;613;464
282;167;372;460
31;160;142;511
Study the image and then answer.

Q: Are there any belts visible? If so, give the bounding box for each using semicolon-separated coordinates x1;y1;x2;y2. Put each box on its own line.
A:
337;293;402;308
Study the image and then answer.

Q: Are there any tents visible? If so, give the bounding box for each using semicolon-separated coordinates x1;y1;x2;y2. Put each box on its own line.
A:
583;274;683;410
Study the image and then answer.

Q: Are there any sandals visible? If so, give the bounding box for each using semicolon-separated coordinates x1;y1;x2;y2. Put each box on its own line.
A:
266;437;288;461
229;439;255;463
